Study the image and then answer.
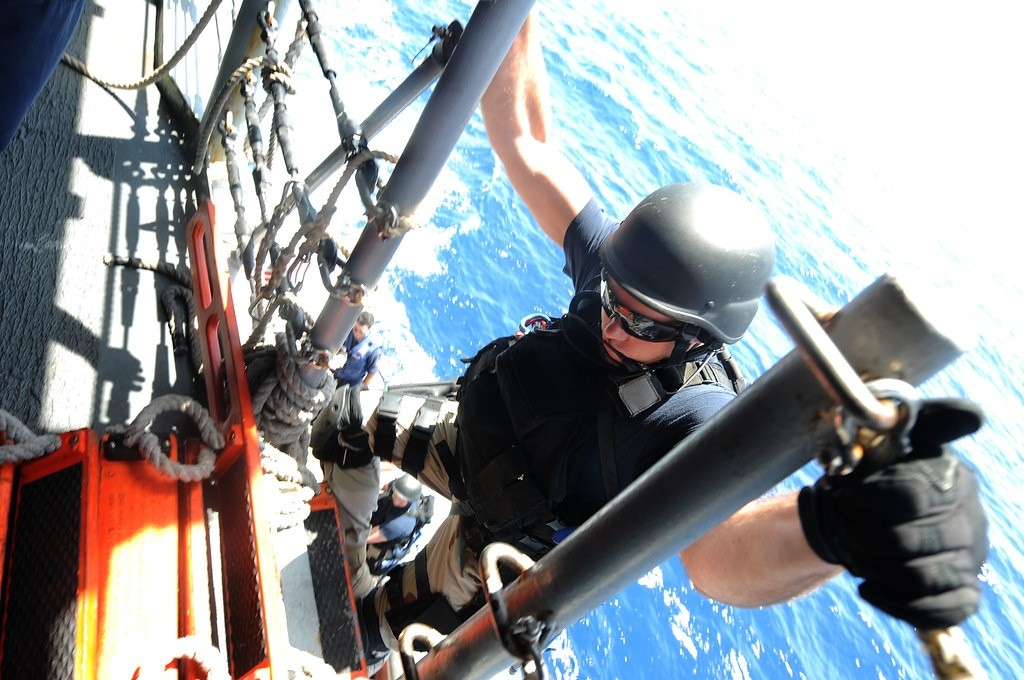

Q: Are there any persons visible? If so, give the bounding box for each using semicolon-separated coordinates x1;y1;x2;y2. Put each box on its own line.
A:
365;470;423;576
327;310;385;392
307;9;990;679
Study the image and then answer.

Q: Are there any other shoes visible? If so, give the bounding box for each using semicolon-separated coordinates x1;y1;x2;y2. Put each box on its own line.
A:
354;565;374;599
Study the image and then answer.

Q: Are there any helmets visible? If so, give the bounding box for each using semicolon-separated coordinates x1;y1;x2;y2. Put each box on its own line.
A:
393;474;419;501
600;182;775;344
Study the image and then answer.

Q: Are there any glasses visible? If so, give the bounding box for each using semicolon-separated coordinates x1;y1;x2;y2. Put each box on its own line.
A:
600;268;684;343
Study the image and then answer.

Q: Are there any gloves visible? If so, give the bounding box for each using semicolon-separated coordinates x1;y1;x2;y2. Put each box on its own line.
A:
797;400;990;629
